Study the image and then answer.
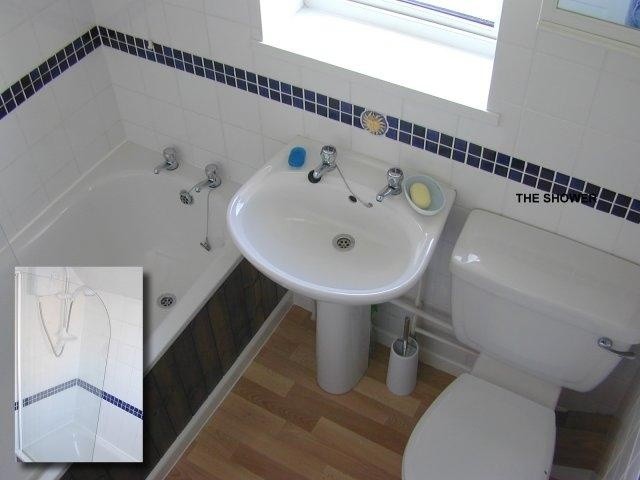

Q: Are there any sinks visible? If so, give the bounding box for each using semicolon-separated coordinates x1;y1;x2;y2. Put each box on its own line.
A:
226;134;456;305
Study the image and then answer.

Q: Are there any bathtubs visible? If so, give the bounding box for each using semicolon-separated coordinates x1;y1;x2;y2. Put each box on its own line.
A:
0;140;294;479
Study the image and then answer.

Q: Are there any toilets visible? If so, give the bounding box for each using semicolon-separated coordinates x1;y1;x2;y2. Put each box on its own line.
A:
398;206;640;480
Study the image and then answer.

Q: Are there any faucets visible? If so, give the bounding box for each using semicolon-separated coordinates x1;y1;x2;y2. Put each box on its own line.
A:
311;145;338;180
194;163;222;194
152;147;179;175
375;167;405;204
